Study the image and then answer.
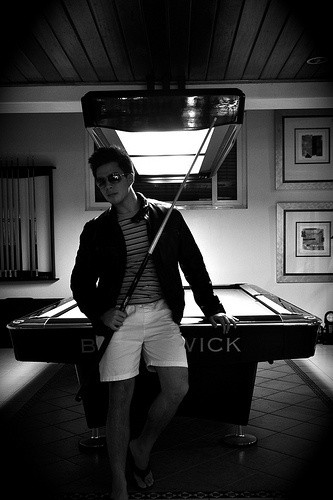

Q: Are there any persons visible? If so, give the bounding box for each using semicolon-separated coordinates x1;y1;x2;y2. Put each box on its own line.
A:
69;145;241;500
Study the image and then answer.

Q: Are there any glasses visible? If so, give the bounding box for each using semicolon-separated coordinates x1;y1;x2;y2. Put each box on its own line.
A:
96;173;127;186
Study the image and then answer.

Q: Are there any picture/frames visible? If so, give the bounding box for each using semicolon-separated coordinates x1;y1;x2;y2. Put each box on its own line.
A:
274;108;332;190
277;201;333;284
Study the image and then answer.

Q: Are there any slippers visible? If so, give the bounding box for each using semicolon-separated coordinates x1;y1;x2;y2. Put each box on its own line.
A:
128;447;153;487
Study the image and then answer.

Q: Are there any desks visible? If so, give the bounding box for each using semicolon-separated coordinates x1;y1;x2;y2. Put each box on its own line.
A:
6;282;323;447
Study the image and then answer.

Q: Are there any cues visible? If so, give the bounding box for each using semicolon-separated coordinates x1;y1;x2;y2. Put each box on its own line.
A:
73;116;219;403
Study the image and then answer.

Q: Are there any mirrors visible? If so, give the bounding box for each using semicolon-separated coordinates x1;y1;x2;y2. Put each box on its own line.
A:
85;111;248;211
